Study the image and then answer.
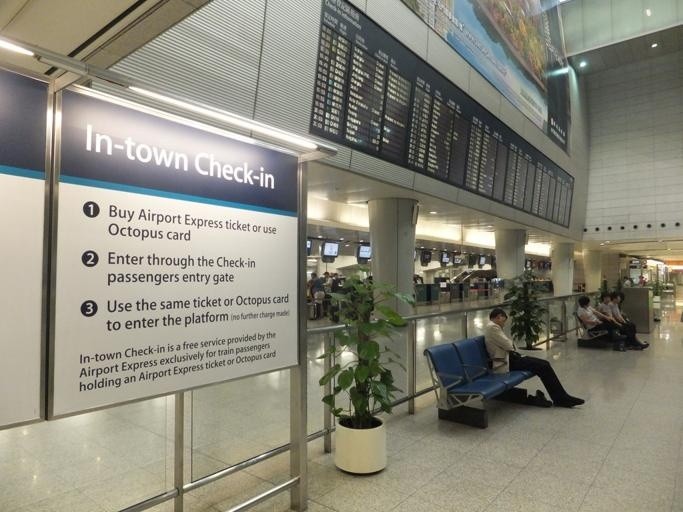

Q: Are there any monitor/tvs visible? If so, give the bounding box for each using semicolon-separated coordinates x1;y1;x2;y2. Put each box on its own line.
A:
421;251;551;268
322;242;339;257
357;245;371;259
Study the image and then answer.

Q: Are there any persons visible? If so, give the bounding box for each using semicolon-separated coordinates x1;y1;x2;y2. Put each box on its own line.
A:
485;308;585;407
577;295;642;352
623;276;631;287
596;291;649;349
308;271;341;318
638;276;644;286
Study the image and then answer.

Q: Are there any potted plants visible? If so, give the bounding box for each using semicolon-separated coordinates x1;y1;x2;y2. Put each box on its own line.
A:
317;267;418;479
502;269;550;361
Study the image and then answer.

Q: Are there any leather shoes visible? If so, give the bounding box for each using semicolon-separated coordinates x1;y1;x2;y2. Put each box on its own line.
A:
526;390;552;407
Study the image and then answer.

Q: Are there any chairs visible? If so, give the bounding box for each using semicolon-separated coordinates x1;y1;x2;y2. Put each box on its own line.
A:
422;334;539;429
